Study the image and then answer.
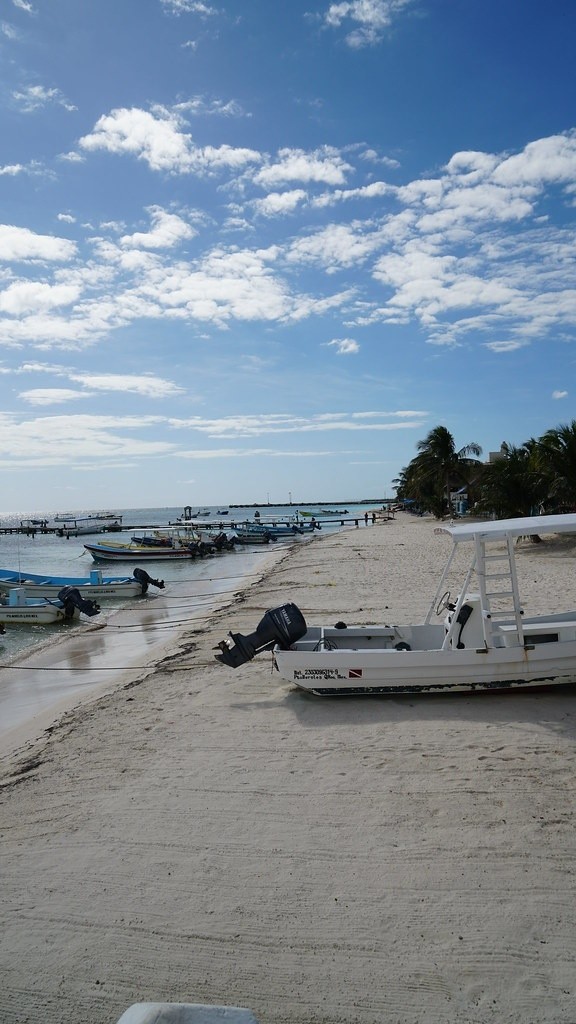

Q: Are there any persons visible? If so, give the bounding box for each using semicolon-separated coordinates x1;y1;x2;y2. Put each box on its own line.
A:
254;511;260;523
312;515;315;525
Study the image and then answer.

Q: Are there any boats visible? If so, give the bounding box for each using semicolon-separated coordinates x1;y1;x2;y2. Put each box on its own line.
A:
216;510;228;515
321;510;349;514
0;566;166;600
53;513;81;522
83;522;320;561
199;508;211;516
299;510;341;517
181;513;198;518
1;585;102;627
210;510;575;696
